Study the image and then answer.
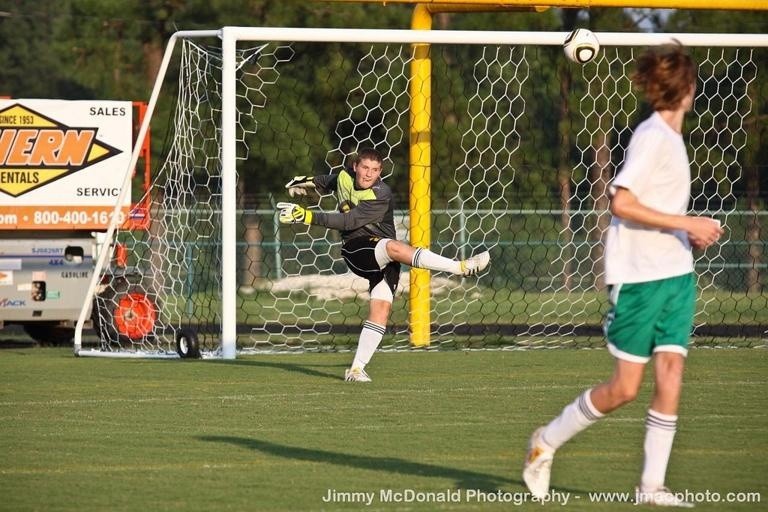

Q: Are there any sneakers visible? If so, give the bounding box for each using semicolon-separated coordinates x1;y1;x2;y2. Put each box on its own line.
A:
344;367;372;382
634;483;696;509
452;250;492;279
521;423;557;500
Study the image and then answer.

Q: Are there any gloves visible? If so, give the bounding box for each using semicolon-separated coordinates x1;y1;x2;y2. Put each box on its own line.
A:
276;201;307;225
285;174;315;198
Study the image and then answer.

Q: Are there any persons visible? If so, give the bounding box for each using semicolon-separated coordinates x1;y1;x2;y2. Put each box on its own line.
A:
520;37;725;509
277;148;490;383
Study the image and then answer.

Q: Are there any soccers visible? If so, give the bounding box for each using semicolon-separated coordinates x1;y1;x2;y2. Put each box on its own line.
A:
563;29;600;64
